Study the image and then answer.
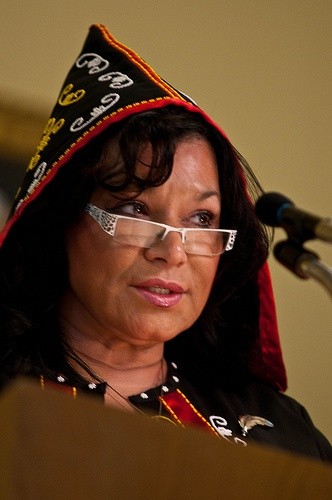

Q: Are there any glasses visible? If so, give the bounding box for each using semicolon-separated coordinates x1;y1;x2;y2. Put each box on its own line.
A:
84;202;237;256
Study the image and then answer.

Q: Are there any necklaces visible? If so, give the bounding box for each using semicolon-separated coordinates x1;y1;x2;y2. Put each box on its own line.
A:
75;358;166;422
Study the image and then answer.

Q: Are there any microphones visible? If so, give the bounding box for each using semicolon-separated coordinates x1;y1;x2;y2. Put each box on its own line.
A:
253;191;332;245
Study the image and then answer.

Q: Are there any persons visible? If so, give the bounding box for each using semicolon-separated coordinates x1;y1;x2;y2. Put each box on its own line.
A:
1;25;331;498
